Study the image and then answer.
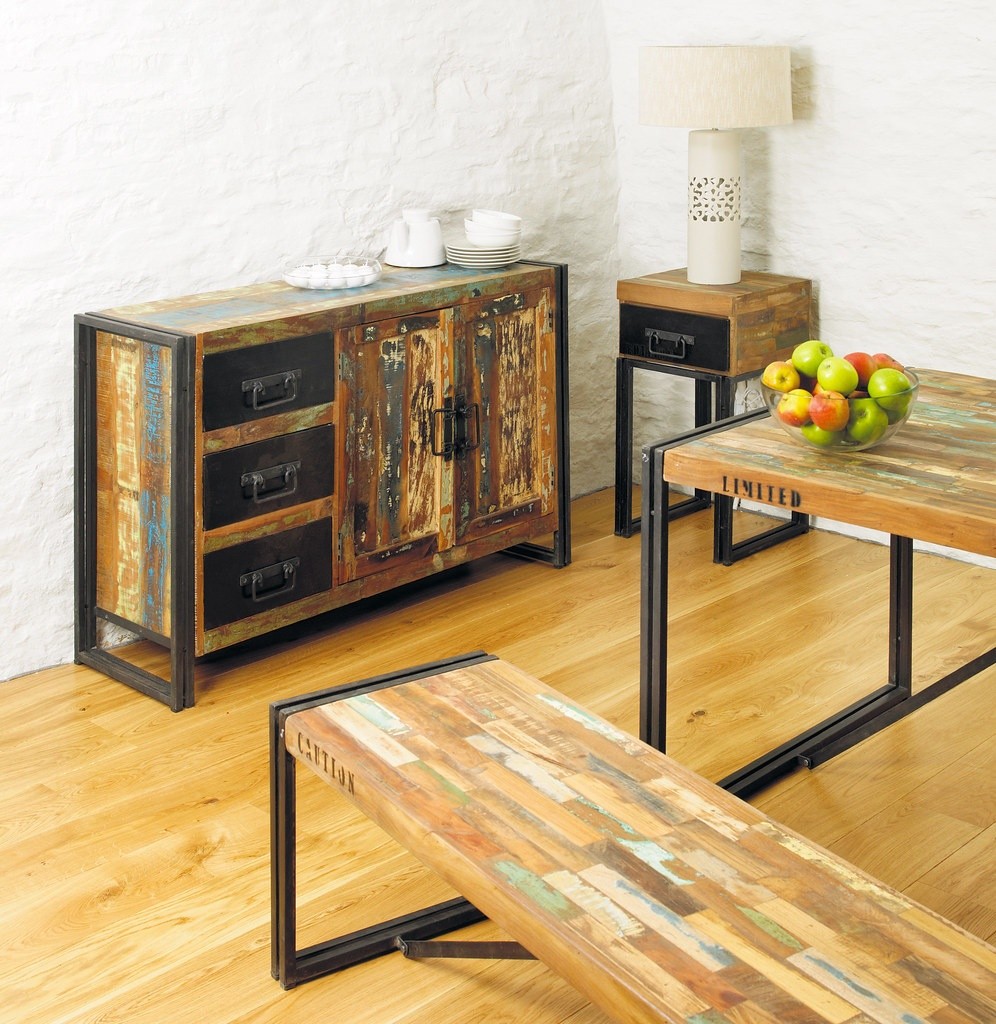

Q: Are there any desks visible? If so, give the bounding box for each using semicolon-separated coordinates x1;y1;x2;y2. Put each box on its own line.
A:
639;366;996;798
616;267;812;567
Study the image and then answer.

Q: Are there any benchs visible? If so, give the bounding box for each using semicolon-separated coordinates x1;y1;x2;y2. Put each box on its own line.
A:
269;652;996;1024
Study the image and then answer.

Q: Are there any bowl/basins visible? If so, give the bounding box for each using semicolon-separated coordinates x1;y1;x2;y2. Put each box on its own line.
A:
760;370;919;452
465;231;522;248
472;209;522;228
464;218;521;233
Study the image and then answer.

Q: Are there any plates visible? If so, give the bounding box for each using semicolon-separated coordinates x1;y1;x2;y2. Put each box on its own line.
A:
281;255;382;289
446;243;522;269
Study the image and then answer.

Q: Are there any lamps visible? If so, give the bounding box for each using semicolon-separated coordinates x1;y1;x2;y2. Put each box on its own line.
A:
637;46;793;285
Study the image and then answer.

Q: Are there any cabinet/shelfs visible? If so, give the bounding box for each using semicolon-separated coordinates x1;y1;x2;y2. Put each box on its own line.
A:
75;260;571;712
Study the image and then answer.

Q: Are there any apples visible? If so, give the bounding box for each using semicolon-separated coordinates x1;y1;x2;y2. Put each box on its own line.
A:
817;356;858;395
801;422;843;447
847;391;868;399
791;340;833;378
887;406;907;425
847;398;888;442
844;352;878;388
868;368;912;409
810;390;849;432
872;353;904;372
763;362;800;394
777;388;814;428
804;376;822;396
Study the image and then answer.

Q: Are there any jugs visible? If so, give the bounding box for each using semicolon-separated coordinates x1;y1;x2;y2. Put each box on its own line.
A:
384;208;448;268
